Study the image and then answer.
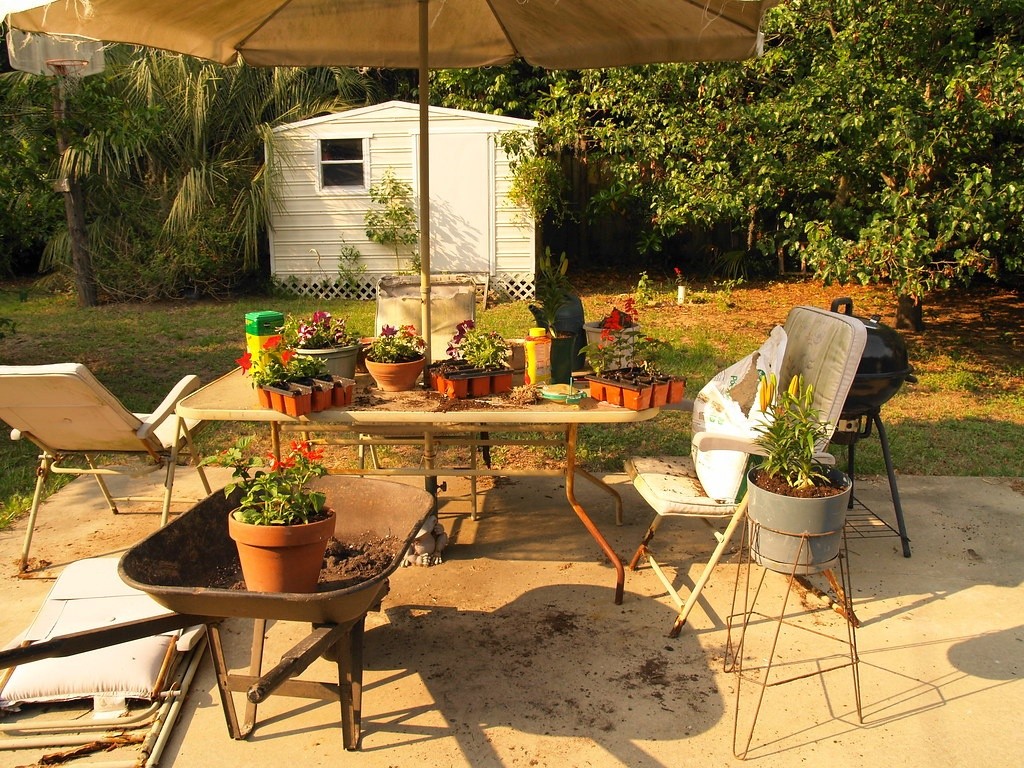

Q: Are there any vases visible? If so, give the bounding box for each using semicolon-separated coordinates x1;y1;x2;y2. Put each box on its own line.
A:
227;501;337;593
677;286;685;306
365;357;426;392
290;343;362;378
584;367;686;411
424;358;514;399
746;464;852;576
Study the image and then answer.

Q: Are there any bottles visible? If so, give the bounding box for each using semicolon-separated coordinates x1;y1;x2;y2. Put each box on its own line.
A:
524;328;552;385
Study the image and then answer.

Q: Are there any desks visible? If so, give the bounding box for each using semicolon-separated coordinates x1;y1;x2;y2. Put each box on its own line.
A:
158;365;659;606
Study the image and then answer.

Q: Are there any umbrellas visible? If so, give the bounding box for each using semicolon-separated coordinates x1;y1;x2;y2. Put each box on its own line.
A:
0;1;776;527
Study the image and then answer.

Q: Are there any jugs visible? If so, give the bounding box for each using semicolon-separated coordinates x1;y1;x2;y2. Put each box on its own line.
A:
528;283;585;335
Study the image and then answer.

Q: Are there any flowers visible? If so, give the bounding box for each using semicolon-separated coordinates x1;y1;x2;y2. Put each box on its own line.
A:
575;295;674;376
747;372;833;489
674;267;683;285
197;435;334;521
273;310;352;347
446;320;519;373
363;324;426;360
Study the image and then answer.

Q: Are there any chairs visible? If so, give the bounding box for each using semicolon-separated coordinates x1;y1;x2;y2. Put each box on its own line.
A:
358;274;477;478
0;362;211;573
624;307;867;639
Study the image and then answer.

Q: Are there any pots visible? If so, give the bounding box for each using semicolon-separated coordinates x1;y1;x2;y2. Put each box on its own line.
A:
831;296;918;414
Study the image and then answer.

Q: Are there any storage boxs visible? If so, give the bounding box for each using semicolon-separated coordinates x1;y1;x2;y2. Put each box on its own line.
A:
245;310;283;371
